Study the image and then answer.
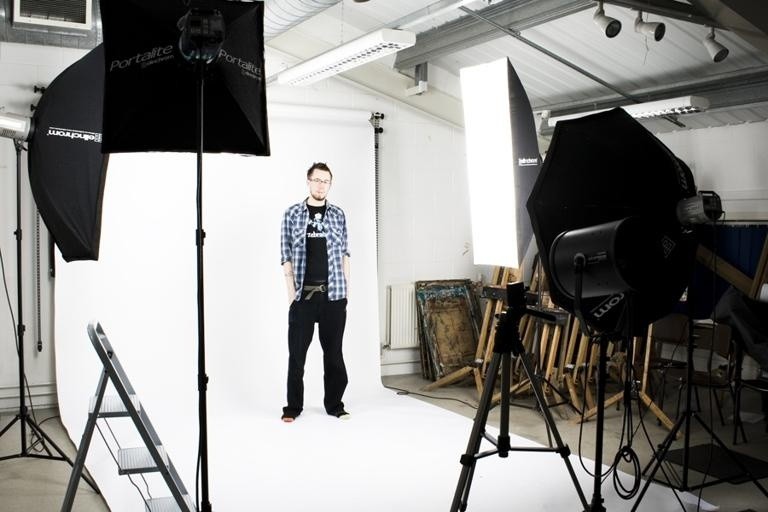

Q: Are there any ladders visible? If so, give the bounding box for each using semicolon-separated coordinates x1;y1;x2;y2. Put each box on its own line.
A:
60;321;197;512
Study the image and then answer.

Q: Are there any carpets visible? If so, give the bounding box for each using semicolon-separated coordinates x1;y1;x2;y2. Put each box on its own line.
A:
649;443;768;485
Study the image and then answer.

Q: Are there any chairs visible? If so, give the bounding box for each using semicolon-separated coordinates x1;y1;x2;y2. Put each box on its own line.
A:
615;312;702;424
656;325;748;446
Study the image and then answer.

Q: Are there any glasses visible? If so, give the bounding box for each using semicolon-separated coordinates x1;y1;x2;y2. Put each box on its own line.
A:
311;178;330;185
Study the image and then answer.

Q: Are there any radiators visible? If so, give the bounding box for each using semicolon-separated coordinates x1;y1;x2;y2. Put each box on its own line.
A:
384;282;421;352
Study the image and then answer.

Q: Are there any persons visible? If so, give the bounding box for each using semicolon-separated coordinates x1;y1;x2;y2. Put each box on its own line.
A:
279;160;354;423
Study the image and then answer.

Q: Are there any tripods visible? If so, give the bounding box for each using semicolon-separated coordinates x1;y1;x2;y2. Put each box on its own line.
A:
0;138;101;494
631;286;768;512
451;319;592;512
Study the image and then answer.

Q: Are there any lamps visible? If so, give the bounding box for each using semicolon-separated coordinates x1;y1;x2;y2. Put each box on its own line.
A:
588;2;731;65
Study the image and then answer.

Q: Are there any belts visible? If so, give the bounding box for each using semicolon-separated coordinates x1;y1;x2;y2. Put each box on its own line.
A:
303;284;328;300
